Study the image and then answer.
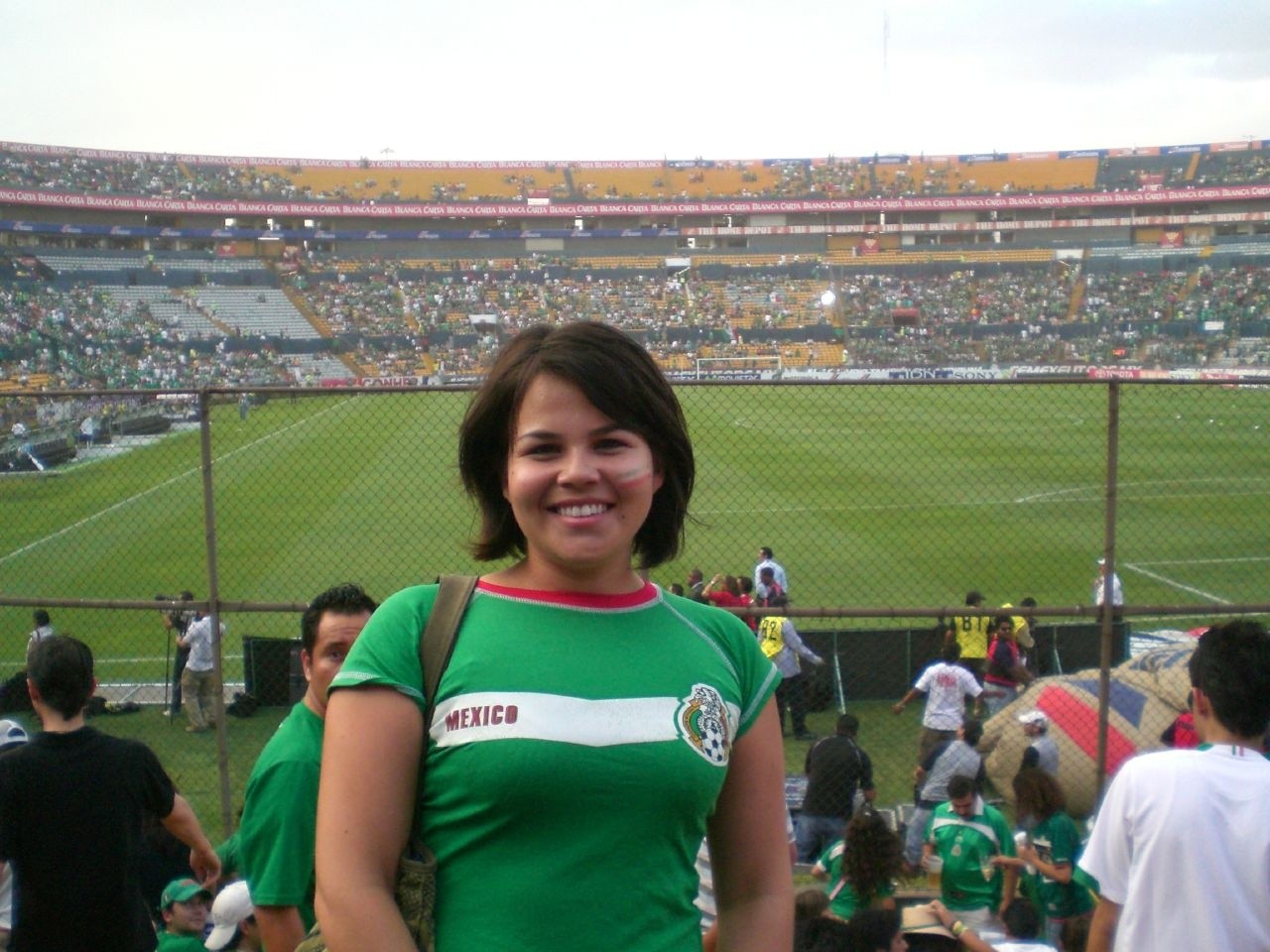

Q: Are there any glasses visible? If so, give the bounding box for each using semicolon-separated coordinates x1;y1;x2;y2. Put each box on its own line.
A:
1002;626;1011;630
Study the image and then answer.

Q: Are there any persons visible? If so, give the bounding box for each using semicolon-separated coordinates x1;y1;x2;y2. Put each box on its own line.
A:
1072;620;1270;952
0;151;1270;952
313;321;795;952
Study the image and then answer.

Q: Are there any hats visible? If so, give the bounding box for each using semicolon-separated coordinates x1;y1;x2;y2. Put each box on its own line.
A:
1019;711;1048;728
1098;558;1107;565
204;881;255;950
966;592;985;605
161;878;203;910
0;720;29;745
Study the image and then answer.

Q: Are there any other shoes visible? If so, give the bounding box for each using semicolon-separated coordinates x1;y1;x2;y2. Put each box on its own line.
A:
899;861;919;877
185;725;196;732
163;710;170;716
795;732;817;741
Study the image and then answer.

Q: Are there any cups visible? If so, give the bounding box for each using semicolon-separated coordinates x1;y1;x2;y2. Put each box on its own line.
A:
1014;831;1038;876
927;856;944;888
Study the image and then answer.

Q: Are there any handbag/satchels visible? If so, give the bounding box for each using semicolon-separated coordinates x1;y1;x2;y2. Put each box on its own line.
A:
294;843;437;952
226;692;260;718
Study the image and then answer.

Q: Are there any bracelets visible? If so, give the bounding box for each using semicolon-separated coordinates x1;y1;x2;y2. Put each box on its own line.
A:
898;700;908;708
950;915;969;938
